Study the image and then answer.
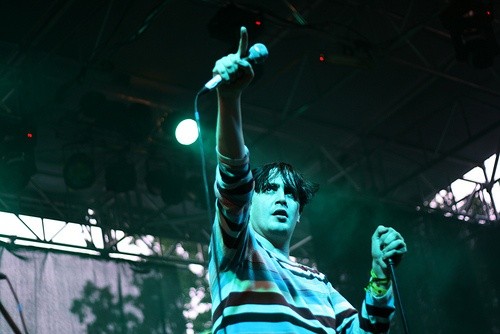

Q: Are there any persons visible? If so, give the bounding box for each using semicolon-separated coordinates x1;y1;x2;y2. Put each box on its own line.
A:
207;25;409;334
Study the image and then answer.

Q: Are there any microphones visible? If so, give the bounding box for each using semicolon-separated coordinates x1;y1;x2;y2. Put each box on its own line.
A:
194;43;270;96
